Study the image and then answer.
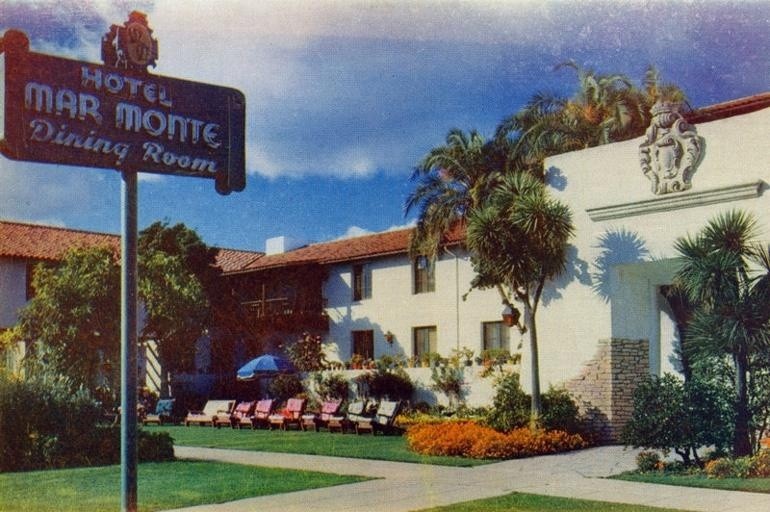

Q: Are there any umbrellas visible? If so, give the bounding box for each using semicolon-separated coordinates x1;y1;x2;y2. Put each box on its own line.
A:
236;352;294;400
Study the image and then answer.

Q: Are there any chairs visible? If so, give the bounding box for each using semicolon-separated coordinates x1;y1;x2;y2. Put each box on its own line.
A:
142;398;176;425
185;398;402;436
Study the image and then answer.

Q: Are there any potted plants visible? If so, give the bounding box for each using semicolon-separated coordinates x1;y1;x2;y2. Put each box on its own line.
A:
351;346;521;369
502;304;521;327
384;330;394;342
